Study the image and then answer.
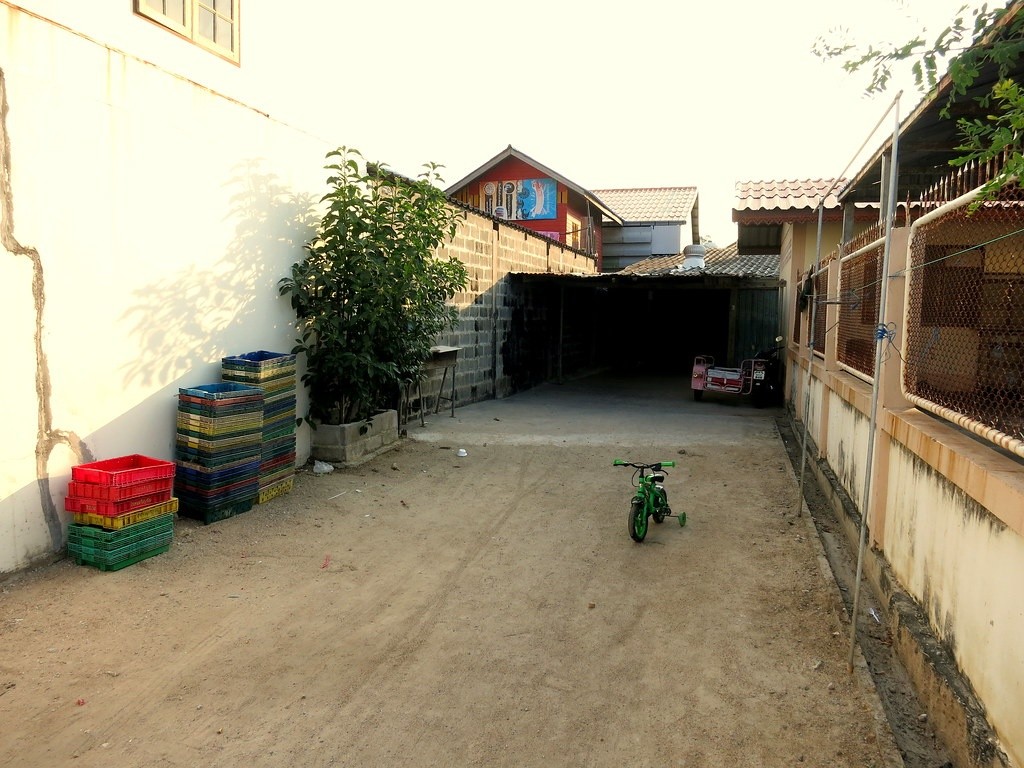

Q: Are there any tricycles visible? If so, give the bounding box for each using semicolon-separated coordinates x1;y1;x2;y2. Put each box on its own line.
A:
614;459;687;543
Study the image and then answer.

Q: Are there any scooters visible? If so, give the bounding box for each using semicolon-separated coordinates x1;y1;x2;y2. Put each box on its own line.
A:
752;336;786;409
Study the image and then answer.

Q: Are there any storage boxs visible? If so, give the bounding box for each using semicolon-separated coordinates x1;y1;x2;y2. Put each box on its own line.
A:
221;351;298;505
64;454;180;573
173;381;266;526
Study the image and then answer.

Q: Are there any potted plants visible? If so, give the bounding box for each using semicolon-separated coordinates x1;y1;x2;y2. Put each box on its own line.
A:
278;145;472;467
